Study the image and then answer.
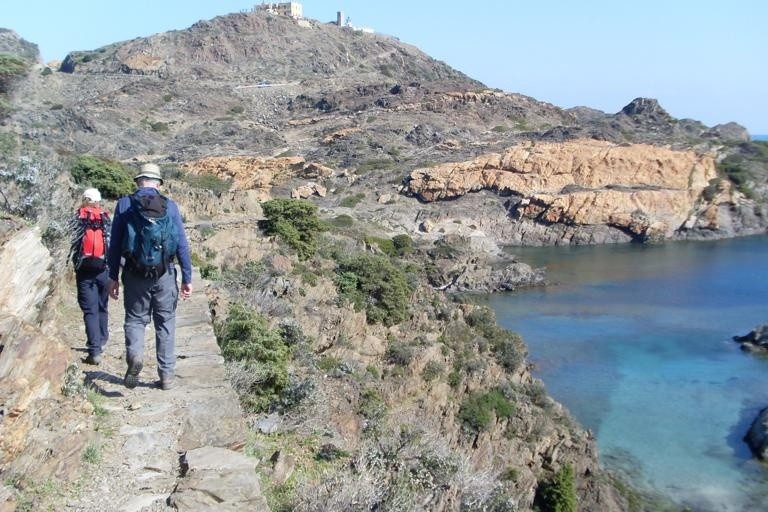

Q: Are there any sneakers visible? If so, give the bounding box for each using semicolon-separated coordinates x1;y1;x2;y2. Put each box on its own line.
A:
86;354;100;364
124;354;143;389
162;375;176;389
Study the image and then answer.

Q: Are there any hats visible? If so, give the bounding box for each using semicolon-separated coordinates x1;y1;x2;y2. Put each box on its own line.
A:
134;163;164;185
82;188;101;203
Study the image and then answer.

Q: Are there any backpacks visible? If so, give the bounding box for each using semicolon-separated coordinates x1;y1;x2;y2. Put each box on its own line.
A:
121;194;180;281
75;207;107;272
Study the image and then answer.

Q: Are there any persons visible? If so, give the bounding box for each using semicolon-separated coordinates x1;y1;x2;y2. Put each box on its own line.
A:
66;188;113;365
109;162;192;391
